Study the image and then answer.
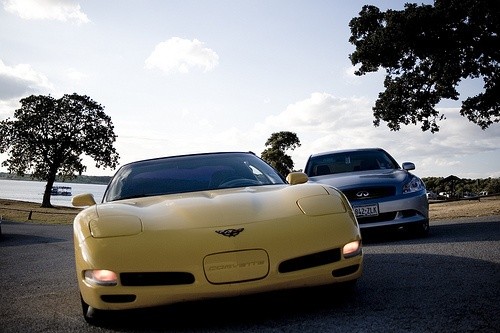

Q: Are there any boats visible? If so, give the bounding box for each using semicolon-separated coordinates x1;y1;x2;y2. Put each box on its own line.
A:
50;186;71;196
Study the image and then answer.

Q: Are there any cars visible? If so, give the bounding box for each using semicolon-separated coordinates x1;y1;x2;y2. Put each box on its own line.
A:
426;191;488;200
304;147;430;237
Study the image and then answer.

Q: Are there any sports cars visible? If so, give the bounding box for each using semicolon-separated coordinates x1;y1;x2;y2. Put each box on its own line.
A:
69;150;363;319
